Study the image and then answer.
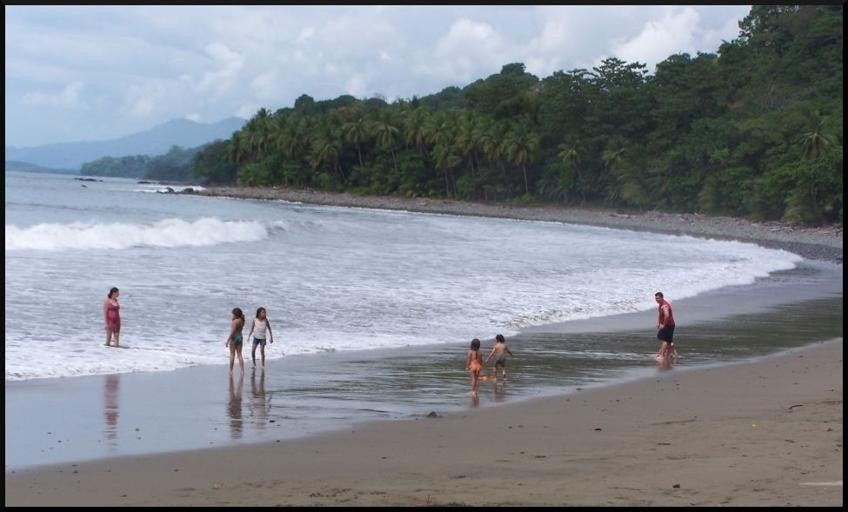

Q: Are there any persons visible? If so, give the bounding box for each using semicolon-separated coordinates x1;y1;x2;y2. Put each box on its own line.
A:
491;385;505;401
657;342;679;363
228;376;246;437
102;286;120;346
654;292;675;359
249;369;272;429
225;307;245;376
470;396;480;408
485;334;513;384
247;307;272;369
465;338;482;396
